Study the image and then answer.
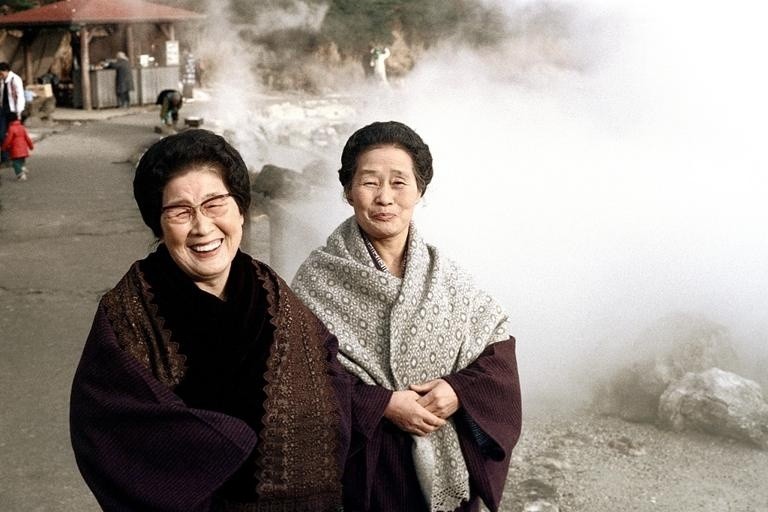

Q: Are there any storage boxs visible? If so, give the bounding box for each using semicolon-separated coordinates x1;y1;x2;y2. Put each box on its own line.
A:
36;82;52;99
24;84;41;98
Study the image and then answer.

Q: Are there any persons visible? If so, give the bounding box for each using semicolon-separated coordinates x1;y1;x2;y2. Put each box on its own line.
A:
361;43;391;80
66;127;358;512
288;117;525;510
150;43;163;64
178;43;198;103
0;110;35;182
154;89;183;127
0;61;26;164
100;51;136;109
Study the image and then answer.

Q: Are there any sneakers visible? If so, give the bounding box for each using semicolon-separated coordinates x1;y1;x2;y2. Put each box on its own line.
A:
17;166;29;182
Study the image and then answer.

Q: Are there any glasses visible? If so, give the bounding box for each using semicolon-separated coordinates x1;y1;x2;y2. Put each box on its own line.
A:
147;192;241;227
